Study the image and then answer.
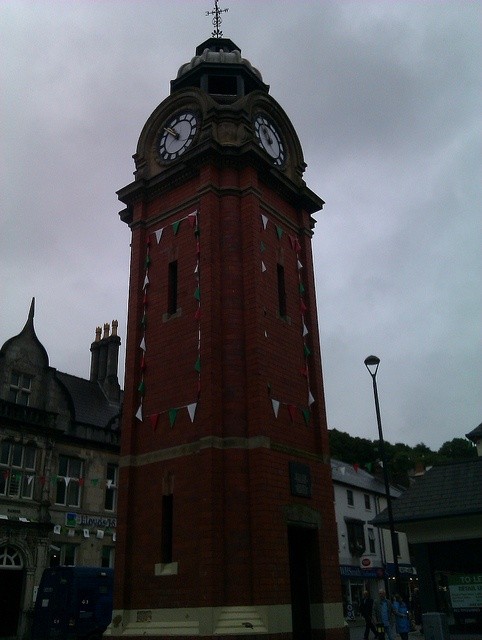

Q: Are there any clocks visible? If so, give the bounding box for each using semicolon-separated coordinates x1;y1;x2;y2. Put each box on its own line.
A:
253;111;290;171
151;103;201;167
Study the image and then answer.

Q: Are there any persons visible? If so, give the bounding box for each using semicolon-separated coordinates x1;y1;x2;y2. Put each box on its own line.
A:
359;591;379;640
372;588;394;640
391;592;416;640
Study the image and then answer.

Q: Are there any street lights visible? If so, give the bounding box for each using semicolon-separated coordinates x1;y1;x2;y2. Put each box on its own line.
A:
363;355;402;592
